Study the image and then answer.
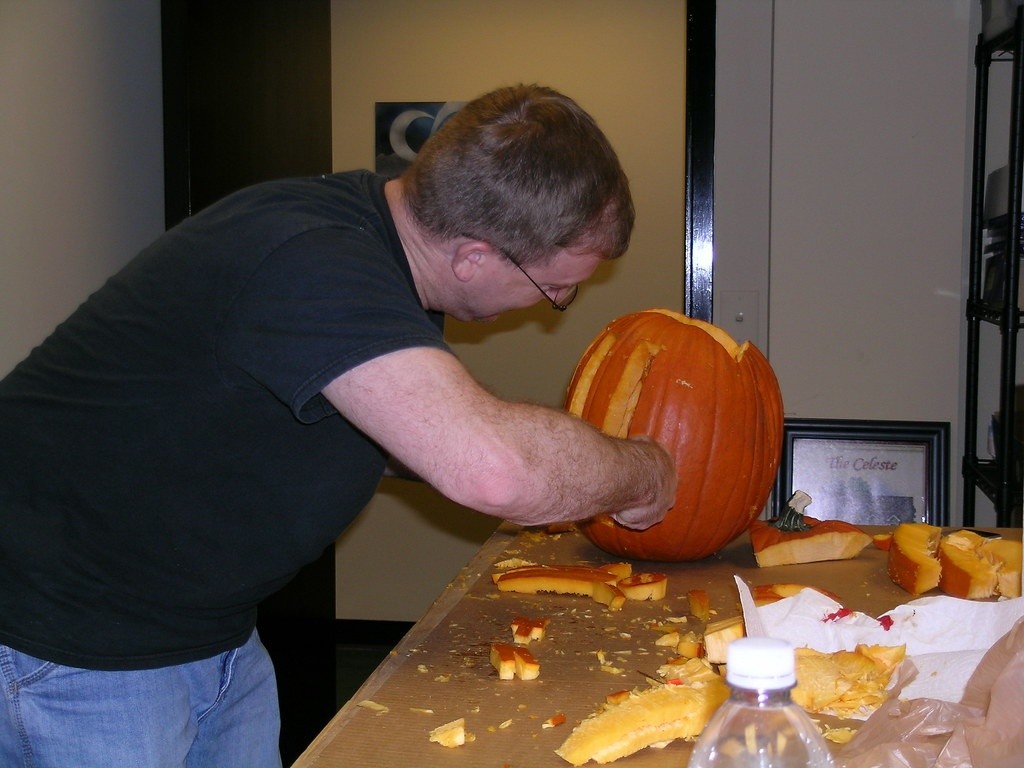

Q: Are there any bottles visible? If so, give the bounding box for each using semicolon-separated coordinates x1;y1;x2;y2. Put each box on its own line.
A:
683;633;840;768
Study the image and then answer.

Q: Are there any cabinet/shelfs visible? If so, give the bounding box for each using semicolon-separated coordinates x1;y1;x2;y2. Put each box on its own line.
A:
960;0;1022;528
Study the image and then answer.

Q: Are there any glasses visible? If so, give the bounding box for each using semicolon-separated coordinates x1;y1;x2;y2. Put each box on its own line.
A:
462;233;578;312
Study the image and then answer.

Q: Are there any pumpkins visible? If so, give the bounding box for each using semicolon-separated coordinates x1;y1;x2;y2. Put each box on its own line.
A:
413;305;1022;762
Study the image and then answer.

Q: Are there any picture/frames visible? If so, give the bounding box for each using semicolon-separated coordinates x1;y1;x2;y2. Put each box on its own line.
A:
768;414;952;530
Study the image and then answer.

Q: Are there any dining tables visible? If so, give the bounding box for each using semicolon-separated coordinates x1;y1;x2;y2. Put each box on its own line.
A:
286;520;1023;766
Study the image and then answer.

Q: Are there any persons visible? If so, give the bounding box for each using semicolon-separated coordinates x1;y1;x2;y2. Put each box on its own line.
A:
0;81;675;765
